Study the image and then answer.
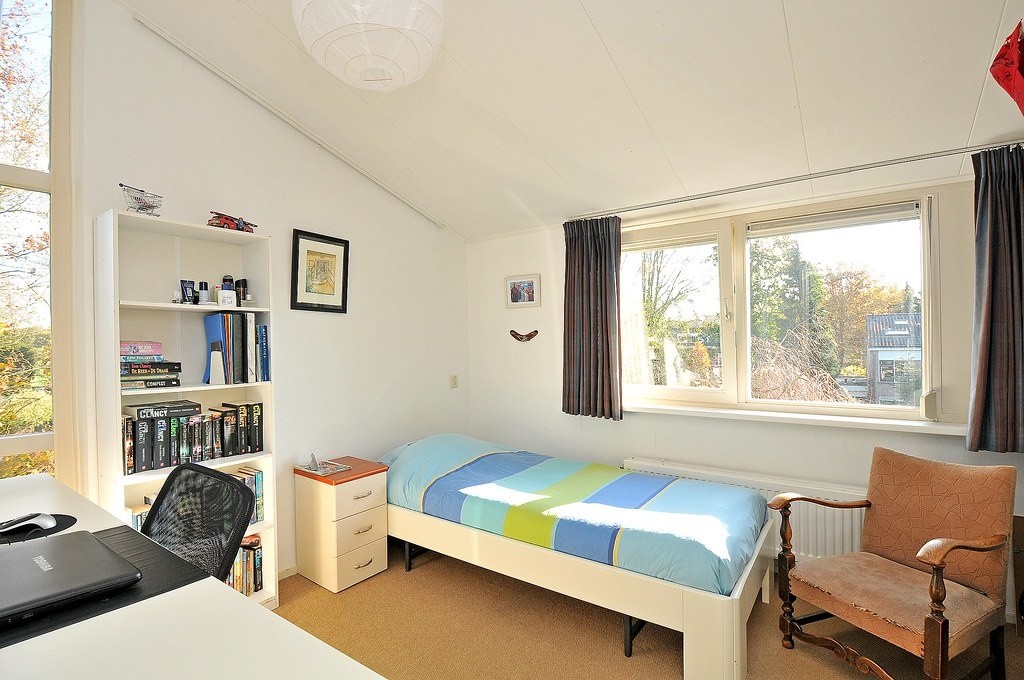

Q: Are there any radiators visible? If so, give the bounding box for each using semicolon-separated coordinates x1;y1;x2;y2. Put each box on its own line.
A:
619;457;869;570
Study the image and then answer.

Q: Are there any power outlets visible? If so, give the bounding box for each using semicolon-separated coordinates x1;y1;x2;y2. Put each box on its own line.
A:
450;375;458;388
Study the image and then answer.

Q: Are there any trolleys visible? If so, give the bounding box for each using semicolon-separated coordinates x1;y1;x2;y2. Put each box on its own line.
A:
119;183;164;217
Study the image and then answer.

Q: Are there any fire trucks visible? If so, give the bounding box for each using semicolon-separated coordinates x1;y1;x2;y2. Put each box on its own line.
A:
207;211;258;233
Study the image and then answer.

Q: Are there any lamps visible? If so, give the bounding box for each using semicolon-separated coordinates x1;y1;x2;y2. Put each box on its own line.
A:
290;0;445;91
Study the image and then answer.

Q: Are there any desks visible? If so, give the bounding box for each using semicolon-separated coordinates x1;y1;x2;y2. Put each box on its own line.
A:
0;472;390;680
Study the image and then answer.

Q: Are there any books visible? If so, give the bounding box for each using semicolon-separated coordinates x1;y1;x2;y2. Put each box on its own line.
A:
297;460;351;477
126;466;264;532
223;536;263;597
121;399;264;476
202;312;269;384
120;340;182;388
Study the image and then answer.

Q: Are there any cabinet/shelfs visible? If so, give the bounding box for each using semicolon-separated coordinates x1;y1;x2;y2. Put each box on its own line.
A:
91;208;282;611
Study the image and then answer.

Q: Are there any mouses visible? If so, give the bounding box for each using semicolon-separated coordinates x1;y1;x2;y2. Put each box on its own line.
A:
0;512;57;537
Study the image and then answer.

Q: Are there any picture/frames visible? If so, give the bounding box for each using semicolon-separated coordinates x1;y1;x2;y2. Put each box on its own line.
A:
289;229;351;314
505;274;542;309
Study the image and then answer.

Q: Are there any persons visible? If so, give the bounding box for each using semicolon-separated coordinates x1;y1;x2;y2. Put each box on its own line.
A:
511;283;534;302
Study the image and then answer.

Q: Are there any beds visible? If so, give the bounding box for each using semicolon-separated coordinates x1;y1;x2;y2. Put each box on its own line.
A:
379;432;777;680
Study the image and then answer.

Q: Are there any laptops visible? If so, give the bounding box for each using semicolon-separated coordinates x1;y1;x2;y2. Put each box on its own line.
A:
0;530;142;624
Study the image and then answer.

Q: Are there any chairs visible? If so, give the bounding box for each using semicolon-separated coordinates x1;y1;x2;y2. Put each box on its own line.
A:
140;462;256;584
767;446;1018;680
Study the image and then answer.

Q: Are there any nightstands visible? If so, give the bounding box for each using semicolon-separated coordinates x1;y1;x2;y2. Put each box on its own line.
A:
292;455;390;595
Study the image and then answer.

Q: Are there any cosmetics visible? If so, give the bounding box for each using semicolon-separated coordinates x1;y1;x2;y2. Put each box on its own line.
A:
174;273;258;307
